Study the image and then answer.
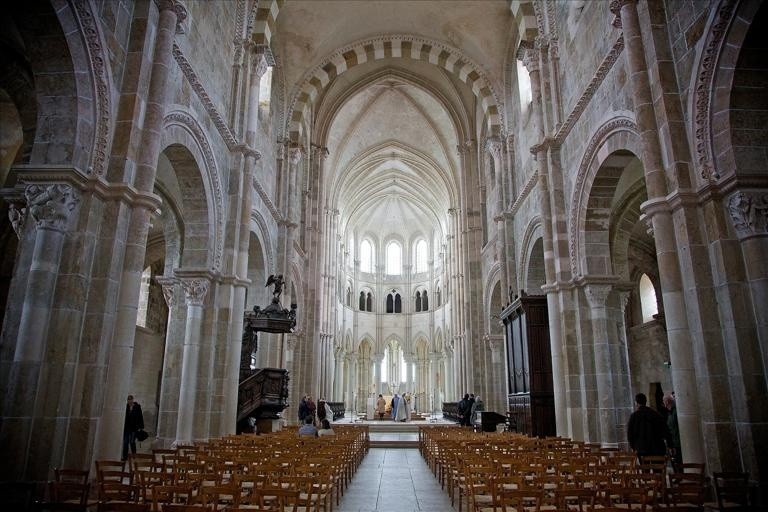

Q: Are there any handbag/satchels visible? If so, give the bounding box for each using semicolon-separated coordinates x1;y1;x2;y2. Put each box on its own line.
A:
136;430;149;441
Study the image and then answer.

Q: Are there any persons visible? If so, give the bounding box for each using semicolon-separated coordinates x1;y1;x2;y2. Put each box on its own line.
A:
662;391;685;483
120;394;144;462
390;392;408;423
317;420;336;438
626;393;676;475
376;393;386;420
455;394;484;428
298;415;317;438
297;394;327;425
